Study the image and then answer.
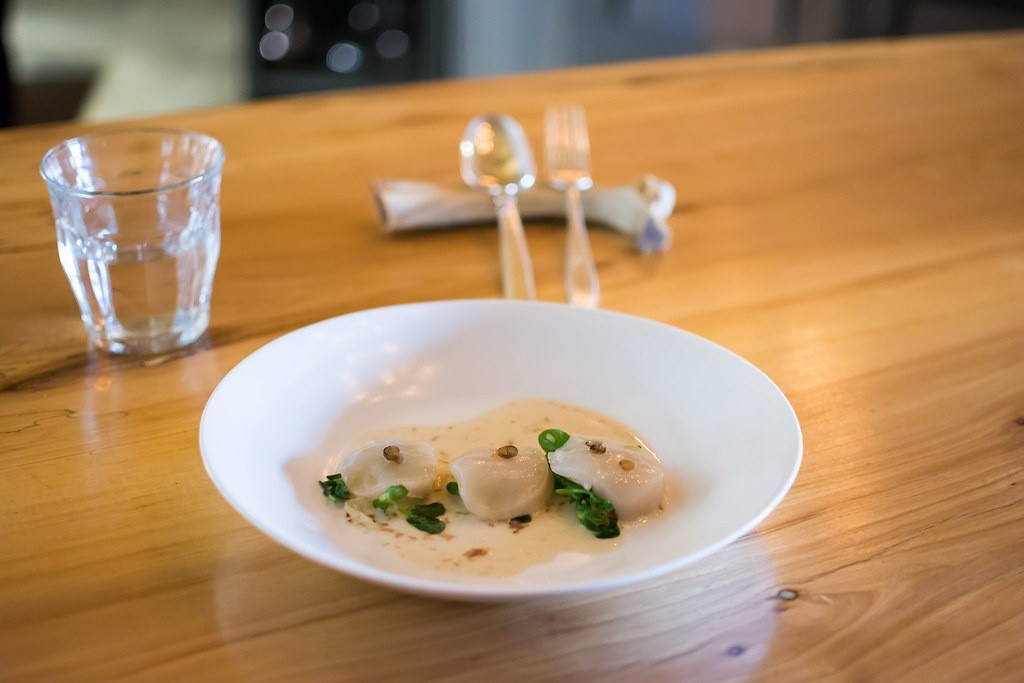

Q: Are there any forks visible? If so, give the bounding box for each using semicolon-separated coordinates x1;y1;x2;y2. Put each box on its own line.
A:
540;104;602;309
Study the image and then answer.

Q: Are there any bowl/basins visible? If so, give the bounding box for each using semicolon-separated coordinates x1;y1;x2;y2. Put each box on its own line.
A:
197;297;803;605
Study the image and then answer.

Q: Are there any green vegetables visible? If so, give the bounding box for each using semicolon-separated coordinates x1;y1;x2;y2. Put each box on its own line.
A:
318;427;619;539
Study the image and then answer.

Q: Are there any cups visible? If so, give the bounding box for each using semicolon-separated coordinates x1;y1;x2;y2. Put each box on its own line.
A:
39;125;226;355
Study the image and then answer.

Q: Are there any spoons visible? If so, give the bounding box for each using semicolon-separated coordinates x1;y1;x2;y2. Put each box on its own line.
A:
459;110;540;296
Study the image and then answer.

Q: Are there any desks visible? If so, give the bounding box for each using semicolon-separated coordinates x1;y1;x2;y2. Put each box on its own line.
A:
0;31;1024;682
